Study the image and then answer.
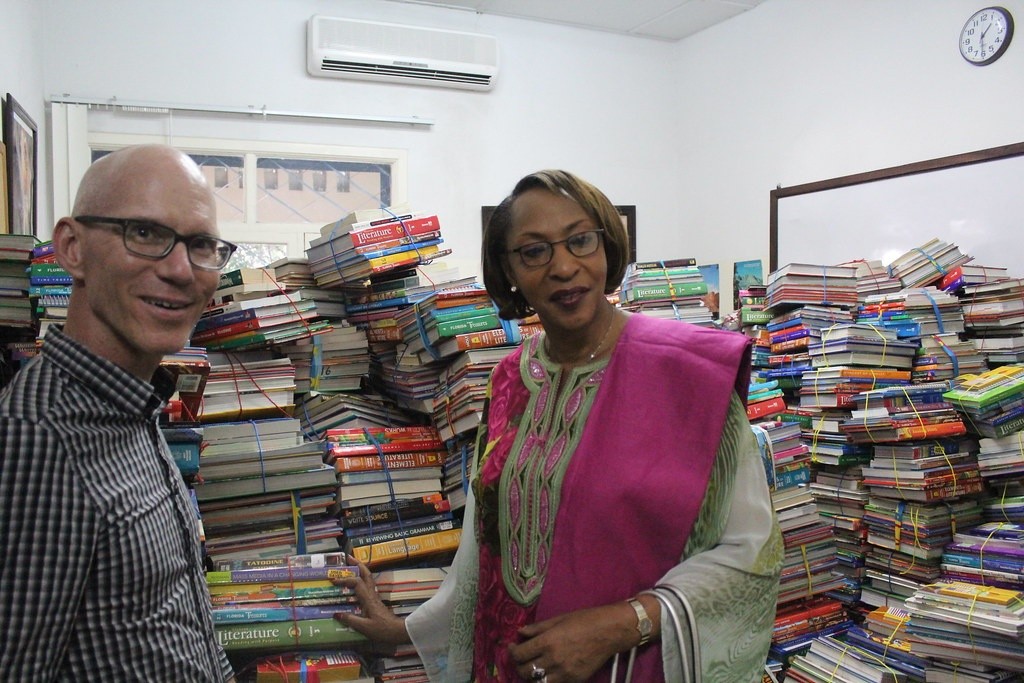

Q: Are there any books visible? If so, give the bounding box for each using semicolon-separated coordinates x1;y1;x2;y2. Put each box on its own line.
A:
151;197;549;682
620;230;1024;683
1;227;75;396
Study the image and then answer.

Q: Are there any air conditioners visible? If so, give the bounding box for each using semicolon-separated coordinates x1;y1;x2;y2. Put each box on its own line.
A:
306;13;498;93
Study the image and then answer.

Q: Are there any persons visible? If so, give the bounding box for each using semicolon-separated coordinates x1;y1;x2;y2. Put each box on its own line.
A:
0;141;239;682
324;167;787;682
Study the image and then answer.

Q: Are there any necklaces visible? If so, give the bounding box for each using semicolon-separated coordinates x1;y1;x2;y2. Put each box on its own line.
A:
546;304;616;374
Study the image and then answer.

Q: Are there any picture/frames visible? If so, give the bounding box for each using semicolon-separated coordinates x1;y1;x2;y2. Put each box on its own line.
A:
482;205;637;275
5;93;38;236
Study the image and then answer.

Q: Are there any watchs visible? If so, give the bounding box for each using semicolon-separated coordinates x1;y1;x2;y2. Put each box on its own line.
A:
625;595;654;646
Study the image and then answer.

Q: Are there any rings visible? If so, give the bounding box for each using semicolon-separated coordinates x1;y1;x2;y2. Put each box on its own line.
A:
374;585;378;592
531;661;545;679
536;675;547;683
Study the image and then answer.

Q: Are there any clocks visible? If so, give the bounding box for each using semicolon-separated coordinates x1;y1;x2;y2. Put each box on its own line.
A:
959;6;1014;66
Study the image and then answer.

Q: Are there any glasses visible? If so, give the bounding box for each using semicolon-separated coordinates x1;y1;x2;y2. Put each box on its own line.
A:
74;216;238;272
507;228;605;267
